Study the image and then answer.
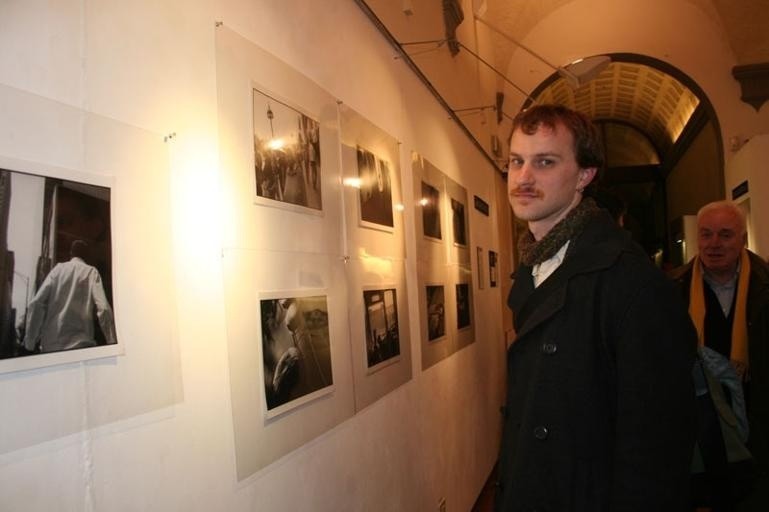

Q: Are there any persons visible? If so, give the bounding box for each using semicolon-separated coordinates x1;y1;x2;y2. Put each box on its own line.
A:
255;129;321;203
666;200;769;511
20;238;116;353
491;104;726;512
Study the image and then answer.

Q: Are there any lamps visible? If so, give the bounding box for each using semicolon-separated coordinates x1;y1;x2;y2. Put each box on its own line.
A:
474;12;612;89
